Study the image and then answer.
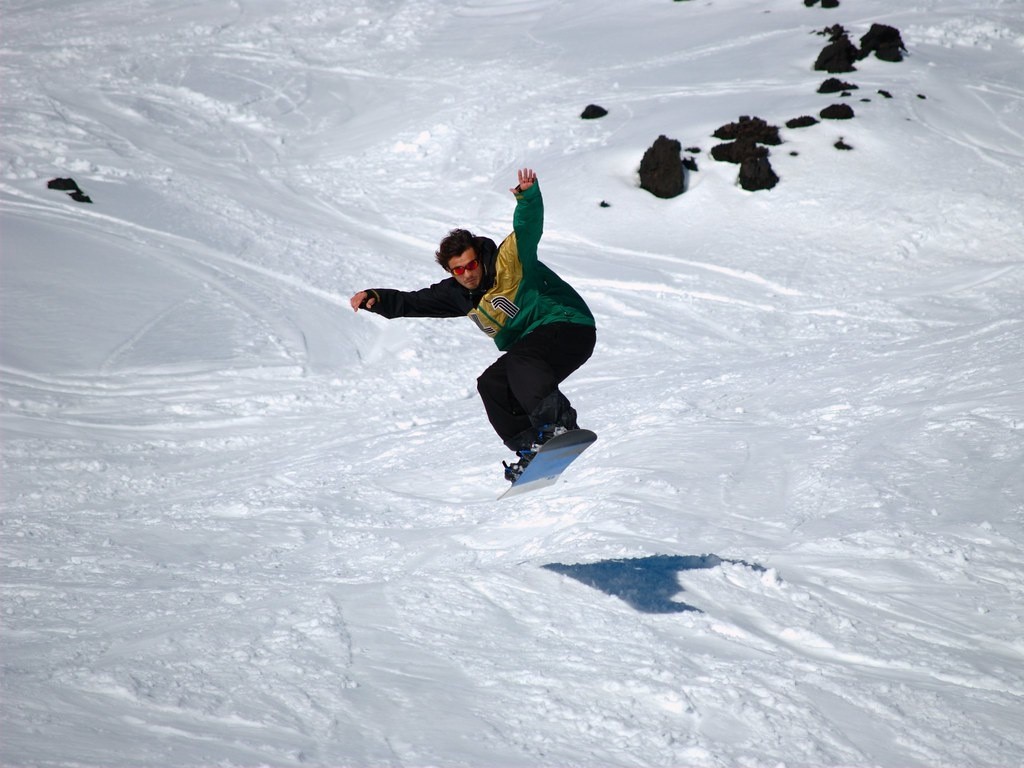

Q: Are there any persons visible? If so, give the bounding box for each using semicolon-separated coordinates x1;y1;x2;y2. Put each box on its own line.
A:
350;167;597;482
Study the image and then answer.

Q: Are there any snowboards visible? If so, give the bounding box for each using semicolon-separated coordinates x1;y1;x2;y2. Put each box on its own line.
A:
495;428;598;500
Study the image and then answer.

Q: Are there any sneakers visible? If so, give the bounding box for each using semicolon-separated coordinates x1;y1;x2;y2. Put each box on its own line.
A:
530;425;581;452
502;450;537;482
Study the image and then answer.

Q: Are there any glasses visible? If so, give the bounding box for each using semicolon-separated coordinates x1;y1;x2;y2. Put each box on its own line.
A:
447;256;479;276
